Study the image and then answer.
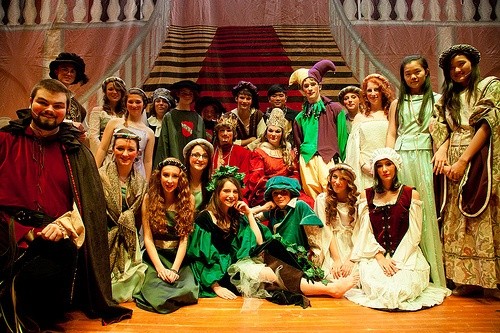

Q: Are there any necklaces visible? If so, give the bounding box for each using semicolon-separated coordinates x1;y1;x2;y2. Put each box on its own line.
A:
212;148;232;174
217;145;233;164
410;94;422;125
118;174;129;183
347;116;354;121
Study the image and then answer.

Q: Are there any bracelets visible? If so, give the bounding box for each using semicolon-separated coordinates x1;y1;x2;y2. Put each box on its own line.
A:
171;269;179;274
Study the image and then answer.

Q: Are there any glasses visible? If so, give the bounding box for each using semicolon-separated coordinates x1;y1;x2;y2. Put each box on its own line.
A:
190;152;209;159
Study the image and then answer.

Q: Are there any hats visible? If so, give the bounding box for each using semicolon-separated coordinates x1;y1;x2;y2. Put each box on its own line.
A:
232;81;257;98
266;108;284;130
338;85;361;102
171;80;201;101
370;147;402;179
102;76;125;90
152;88;172;107
264;176;301;202
289;60;336;88
49;52;86;85
183;138;215;159
438;44;482;69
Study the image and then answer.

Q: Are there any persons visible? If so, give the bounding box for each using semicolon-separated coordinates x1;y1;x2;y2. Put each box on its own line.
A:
88;44;500;313
49;52;89;133
0;78;134;333
